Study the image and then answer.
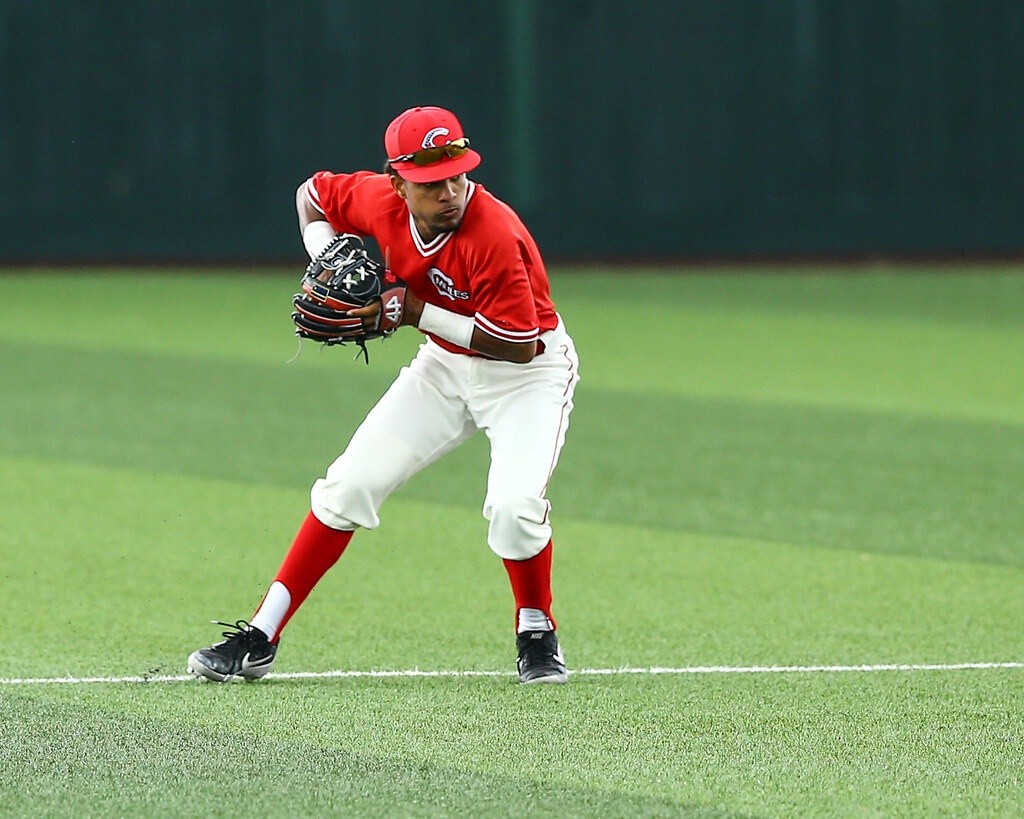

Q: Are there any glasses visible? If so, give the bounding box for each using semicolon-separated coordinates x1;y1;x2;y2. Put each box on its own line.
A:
388;137;471;166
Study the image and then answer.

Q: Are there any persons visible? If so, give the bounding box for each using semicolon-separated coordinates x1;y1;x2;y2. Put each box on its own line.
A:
187;104;580;685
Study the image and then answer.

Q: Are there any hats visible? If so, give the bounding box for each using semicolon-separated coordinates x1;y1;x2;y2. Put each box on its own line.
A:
384;106;481;183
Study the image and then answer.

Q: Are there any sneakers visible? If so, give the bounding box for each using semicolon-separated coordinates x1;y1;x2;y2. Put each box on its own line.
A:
516;631;566;683
188;620;280;682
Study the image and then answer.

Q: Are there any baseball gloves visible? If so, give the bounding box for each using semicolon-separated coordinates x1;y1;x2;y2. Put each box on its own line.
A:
291;233;408;344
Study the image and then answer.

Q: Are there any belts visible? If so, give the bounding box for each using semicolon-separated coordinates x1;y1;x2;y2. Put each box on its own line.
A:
469;339;545;360
